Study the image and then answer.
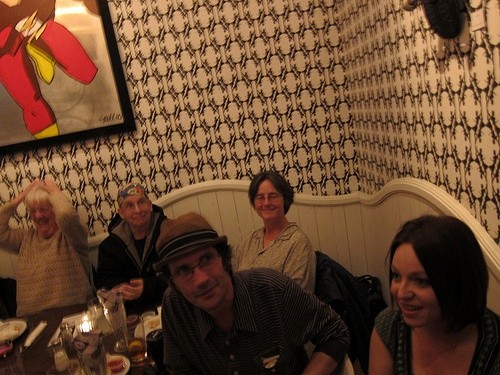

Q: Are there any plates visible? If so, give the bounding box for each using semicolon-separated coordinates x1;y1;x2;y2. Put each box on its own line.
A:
0;321;28;339
81;354;131;375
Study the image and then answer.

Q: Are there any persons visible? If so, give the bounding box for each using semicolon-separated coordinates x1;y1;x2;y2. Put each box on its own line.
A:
231;170;316;369
369;215;500;375
153;212;350;375
0;179;95;316
97;182;169;314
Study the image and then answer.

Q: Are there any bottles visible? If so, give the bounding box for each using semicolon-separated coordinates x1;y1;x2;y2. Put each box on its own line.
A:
51;337;70;372
59;322;74;354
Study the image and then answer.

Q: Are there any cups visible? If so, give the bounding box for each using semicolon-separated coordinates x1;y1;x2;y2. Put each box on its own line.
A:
147;330;167;374
103;299;131;352
122;315;149;362
87;297;114;338
75;339;108;375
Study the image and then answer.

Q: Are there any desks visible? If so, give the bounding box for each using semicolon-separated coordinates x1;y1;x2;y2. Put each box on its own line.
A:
0;306;139;375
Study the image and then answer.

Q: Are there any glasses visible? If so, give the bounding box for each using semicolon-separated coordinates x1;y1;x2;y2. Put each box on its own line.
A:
170;253;223;284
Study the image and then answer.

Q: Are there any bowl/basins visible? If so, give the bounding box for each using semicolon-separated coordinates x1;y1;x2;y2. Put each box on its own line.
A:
134;315;162;337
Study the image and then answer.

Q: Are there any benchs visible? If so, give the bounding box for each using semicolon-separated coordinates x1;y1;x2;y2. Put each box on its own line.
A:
0;179;500;315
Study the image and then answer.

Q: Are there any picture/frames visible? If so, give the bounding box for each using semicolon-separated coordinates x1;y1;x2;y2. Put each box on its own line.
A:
0;0;137;155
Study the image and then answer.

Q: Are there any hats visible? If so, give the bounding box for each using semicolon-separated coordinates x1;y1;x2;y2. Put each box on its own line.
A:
151;211;228;272
